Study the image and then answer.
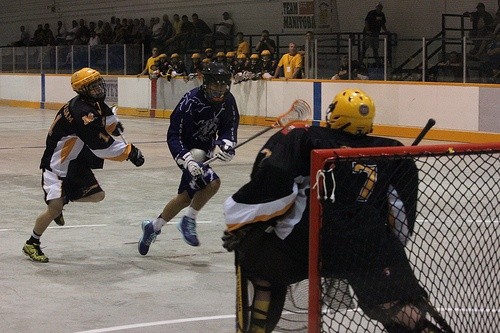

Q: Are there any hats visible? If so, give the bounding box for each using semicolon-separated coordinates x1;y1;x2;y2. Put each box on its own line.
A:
476;3;485;8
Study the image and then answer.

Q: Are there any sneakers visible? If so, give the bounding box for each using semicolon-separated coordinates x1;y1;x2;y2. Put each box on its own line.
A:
137;219;162;256
22;240;49;263
176;215;200;246
44;196;65;226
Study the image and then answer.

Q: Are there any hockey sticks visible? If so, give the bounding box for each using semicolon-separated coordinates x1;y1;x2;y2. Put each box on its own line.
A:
111;104;128;146
411;118;435;148
203;99;312;166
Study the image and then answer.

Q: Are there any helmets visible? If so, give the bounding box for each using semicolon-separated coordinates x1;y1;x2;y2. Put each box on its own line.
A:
261;50;271;55
71;67;107;101
202;60;233;103
250;54;259;59
191;53;201;59
216;52;224;57
171;53;178;58
326;87;376;134
205;48;212;53
202;58;211;63
159;53;167;58
226;52;235;58
237;54;246;58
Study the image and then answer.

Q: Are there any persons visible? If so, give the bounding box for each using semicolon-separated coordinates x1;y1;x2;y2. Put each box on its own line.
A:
221;88;454;333
362;2;389;65
137;62;240;255
331;56;362;80
462;0;500;84
19;2;316;82
21;68;144;264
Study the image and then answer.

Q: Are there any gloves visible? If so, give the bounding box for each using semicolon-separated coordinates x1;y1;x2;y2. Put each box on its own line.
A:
127;144;145;167
112;122;124;136
187;160;205;177
217;144;236;164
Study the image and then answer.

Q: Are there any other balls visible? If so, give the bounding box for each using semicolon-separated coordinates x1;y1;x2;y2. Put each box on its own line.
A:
191;148;205;162
